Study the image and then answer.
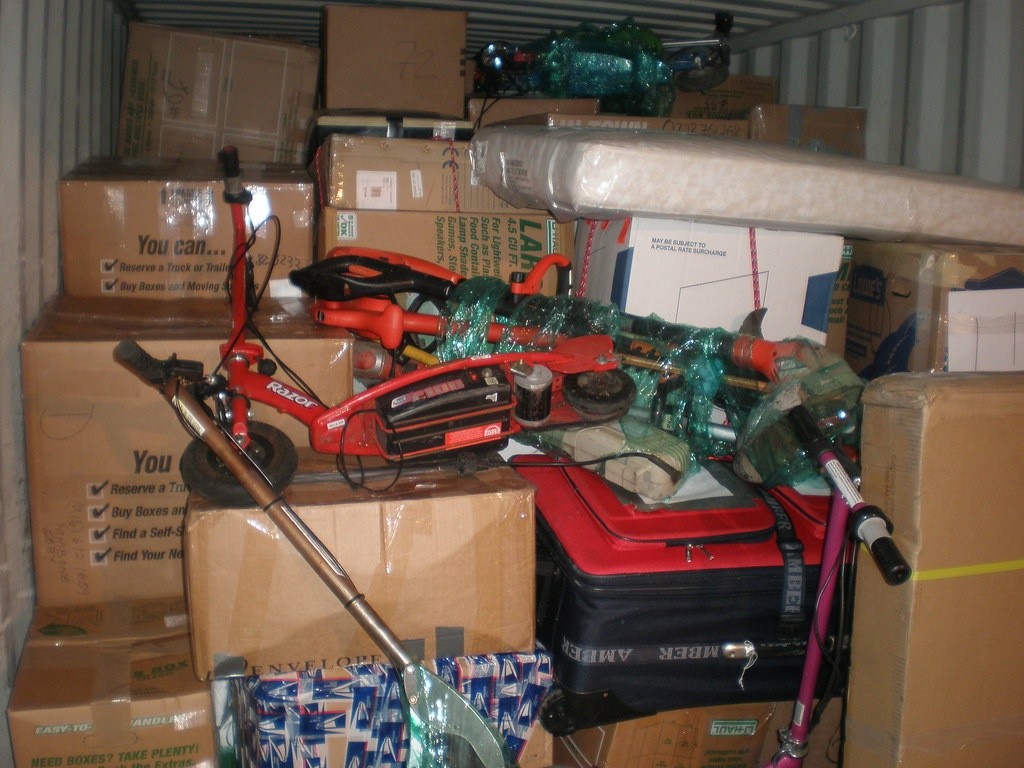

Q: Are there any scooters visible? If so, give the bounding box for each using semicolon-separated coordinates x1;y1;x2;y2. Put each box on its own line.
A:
771;408;912;768
177;140;635;512
110;339;517;766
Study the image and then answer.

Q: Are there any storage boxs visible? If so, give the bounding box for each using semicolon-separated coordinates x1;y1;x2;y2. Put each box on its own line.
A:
8;6;1020;767
